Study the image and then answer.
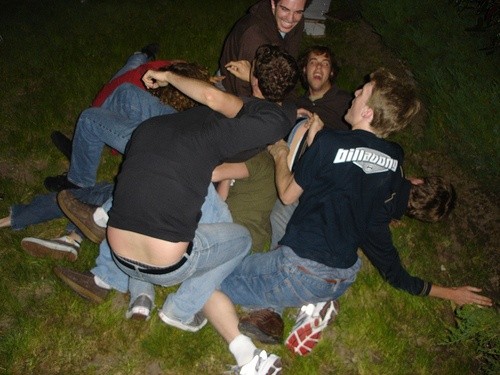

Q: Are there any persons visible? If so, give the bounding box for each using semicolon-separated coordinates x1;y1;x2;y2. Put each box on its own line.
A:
0;0;455;344
203;67;492;375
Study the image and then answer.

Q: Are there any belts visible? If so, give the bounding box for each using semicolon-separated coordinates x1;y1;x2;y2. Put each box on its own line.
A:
294;263;349;285
112;240;194;276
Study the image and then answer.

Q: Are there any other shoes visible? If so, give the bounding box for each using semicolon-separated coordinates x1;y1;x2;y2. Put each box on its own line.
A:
21;235;79;261
50;131;72;159
54;267;110;305
58;188;107;245
125;294;153;321
158;308;208;332
45;171;83;191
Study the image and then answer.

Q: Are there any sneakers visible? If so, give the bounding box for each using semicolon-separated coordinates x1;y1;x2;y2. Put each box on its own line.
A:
227;348;283;375
284;298;341;356
238;306;285;345
